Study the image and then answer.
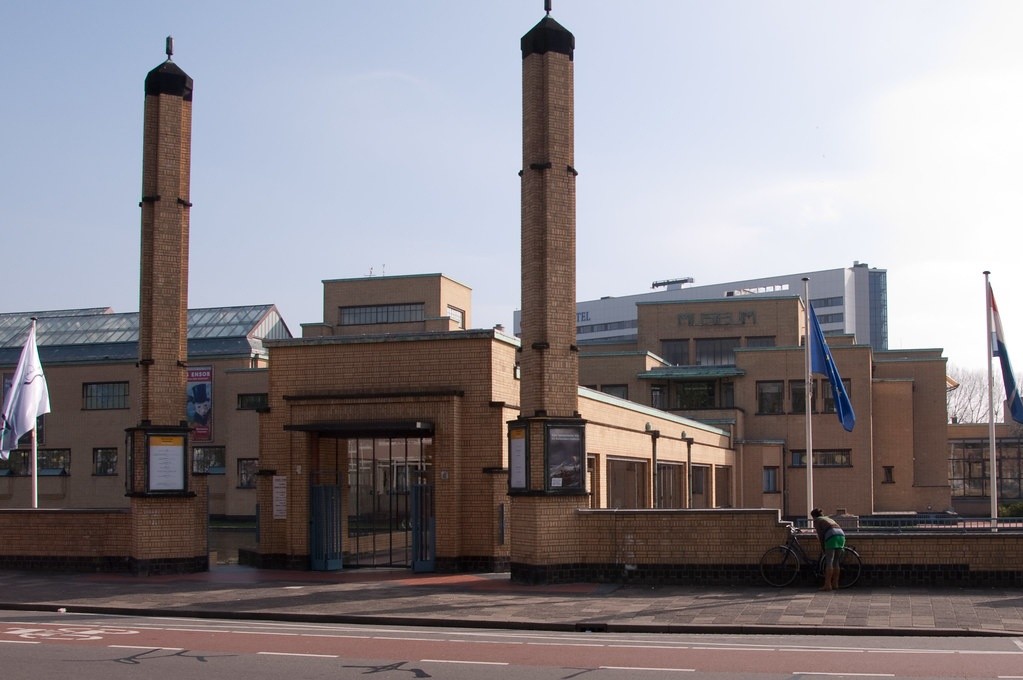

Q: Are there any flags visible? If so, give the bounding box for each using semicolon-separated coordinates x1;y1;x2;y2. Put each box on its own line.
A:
989;282;1023;424
0;320;51;461
808;298;857;433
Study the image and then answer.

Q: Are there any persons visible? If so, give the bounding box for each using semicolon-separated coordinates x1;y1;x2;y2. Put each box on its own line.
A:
811;508;845;590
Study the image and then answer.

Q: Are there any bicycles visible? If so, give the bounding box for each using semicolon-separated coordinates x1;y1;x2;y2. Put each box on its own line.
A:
760;525;862;590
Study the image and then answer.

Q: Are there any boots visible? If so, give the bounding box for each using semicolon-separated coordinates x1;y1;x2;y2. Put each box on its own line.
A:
818;575;833;591
831;576;838;589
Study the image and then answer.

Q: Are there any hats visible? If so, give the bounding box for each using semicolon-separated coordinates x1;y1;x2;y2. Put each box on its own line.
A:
192;383;210;404
